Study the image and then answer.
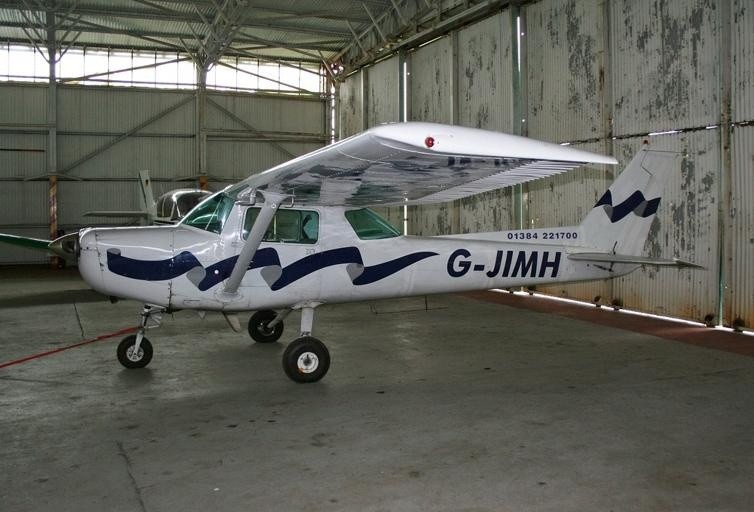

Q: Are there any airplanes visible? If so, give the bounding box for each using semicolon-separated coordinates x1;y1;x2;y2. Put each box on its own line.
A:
47;121;708;386
0;170;383;315
